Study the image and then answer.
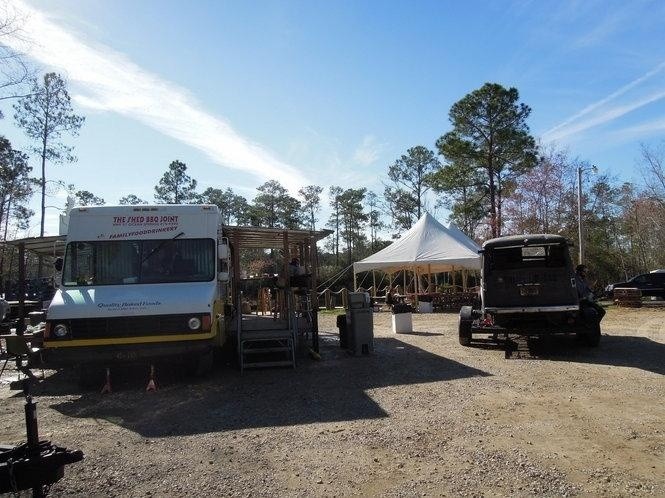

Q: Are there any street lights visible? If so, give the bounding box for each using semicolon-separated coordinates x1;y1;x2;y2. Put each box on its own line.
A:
578;165;598;265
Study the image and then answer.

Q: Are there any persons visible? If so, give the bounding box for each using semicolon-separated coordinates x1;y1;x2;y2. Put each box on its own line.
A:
278;257;301;277
393;289;399;295
385;289;397;306
576;265;606;323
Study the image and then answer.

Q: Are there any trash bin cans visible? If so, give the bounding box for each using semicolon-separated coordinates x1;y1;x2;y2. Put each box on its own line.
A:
337;315;347;347
391;304;412;334
28;311;45;326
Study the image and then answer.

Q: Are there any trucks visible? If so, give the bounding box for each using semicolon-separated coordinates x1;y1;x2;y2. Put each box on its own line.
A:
457;233;603;352
42;202;235;379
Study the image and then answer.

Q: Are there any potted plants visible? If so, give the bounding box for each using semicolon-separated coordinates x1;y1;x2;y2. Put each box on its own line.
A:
393;303;417;334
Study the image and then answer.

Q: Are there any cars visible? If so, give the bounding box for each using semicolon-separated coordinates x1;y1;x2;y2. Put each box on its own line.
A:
604;273;665;300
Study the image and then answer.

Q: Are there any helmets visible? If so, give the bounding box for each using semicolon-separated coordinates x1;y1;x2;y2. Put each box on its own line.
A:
576;265;587;271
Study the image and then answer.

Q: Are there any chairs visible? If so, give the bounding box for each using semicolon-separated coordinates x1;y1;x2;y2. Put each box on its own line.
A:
0;334;45;380
